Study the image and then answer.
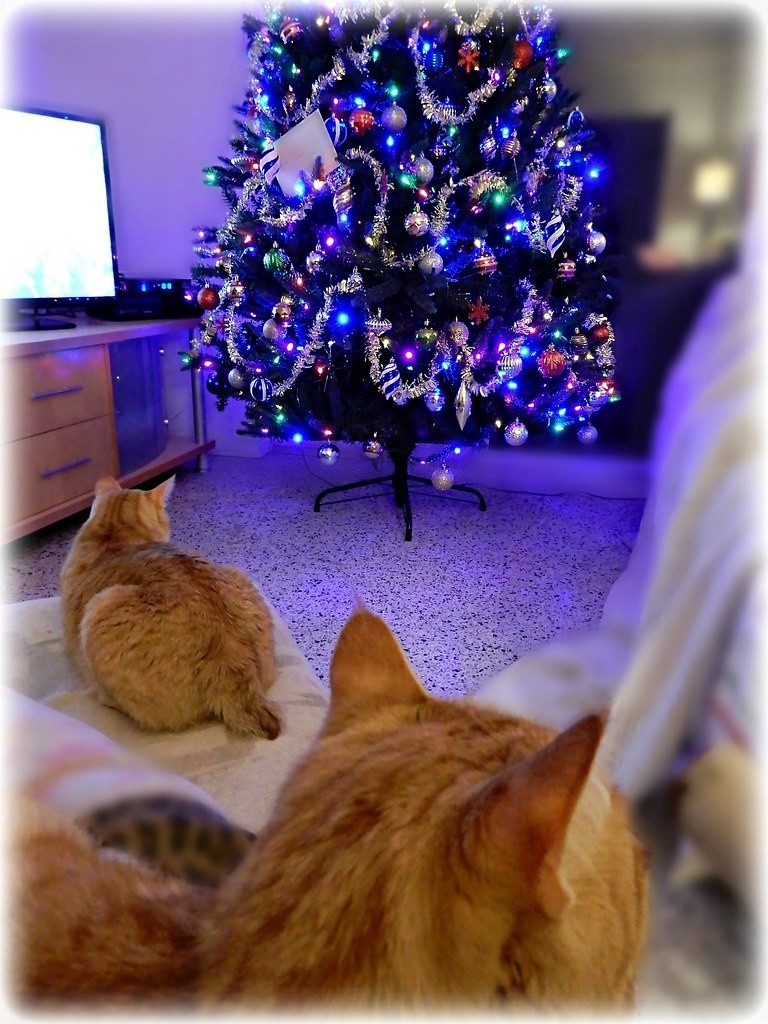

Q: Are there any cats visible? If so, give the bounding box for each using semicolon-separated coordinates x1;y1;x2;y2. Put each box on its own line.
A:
6;592;653;1016
59;471;283;740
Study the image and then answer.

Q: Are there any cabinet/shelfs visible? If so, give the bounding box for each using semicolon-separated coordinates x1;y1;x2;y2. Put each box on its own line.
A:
0;324;217;556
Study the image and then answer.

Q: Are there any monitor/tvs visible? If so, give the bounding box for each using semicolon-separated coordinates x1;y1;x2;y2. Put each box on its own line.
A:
0;106;120;329
